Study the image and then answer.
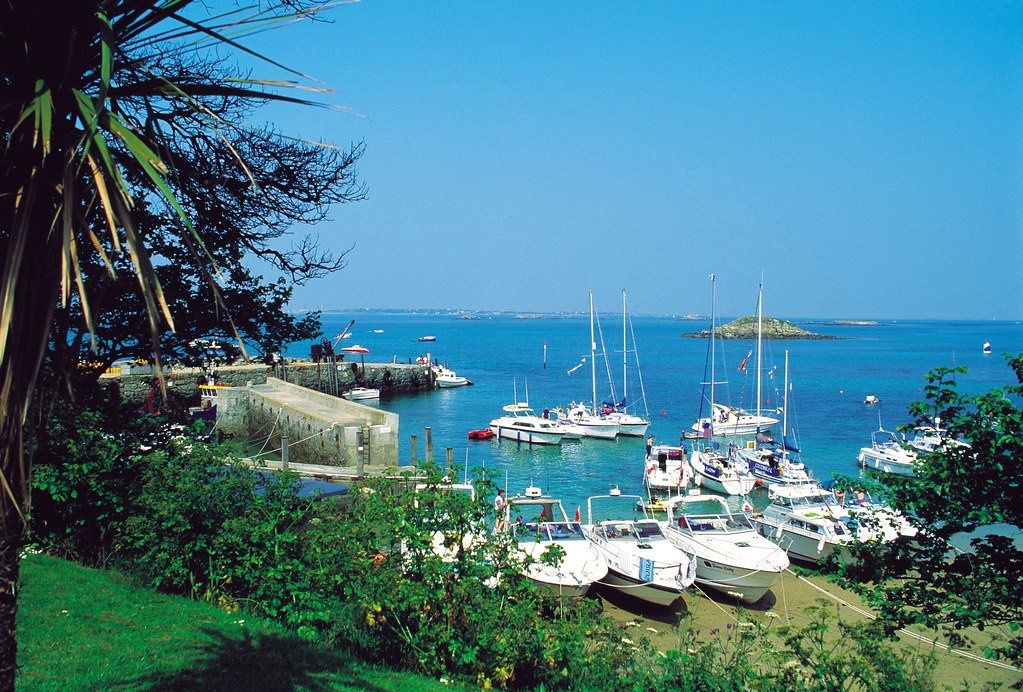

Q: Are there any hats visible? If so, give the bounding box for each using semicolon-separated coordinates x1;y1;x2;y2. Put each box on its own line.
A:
858;488;864;492
841;487;846;493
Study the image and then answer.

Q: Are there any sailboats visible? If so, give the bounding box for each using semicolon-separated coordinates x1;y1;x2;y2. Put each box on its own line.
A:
736;350;815;489
551;288;621;440
983;342;992;355
692;272;779;435
601;289;652;438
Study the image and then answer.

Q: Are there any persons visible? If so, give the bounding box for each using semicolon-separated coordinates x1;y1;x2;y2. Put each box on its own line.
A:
646;435;653;459
439;365;442;373
606;519;618;534
835;488;845;502
509;516;522;530
719;410;727;423
570;401;575;409
416;356;427;365
850;484;866;504
578;402;585;417
658;451;667;472
542;407;550;419
495;490;514;531
768;454;775;467
601;404;611;415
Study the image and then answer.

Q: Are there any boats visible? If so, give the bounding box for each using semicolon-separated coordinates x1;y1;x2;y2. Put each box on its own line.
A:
644;446;693;492
657;491;794;604
760;483;931;573
468;428;492;441
333;334;350;338
371;329;383;333
905;418;973;457
342;387;380;401
577;485;698;608
418;336;436;341
488;469;609;607
400;448;496;574
342;345;369;353
435;374;471;387
690;442;757;497
487;378;567;445
855;410;931;478
